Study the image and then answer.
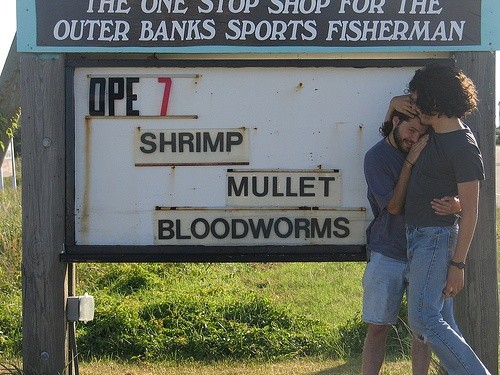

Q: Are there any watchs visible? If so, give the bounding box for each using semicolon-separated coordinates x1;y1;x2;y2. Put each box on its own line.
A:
451;261;465;269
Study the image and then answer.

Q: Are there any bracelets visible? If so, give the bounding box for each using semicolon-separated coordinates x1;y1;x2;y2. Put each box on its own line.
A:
405;159;414;167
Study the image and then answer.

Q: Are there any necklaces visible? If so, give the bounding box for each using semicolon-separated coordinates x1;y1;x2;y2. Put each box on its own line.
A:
388;134;398;151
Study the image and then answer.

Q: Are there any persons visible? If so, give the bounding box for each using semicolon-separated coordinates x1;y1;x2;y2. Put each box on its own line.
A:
361;110;432;375
385;64;493;375
